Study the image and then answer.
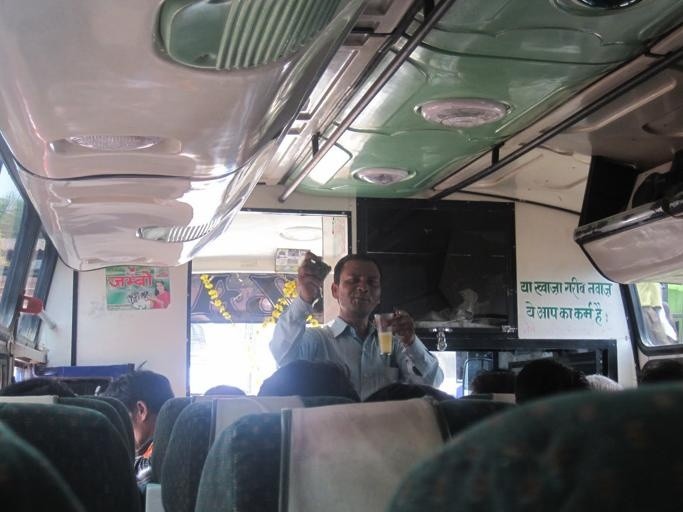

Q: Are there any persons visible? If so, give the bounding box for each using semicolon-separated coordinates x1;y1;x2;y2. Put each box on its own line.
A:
100;371;177;500
146;280;170;309
1;374;79;396
268;250;439;402
203;357;682;440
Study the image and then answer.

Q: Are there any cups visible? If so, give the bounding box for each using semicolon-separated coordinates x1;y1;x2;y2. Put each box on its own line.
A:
374;312;395;355
